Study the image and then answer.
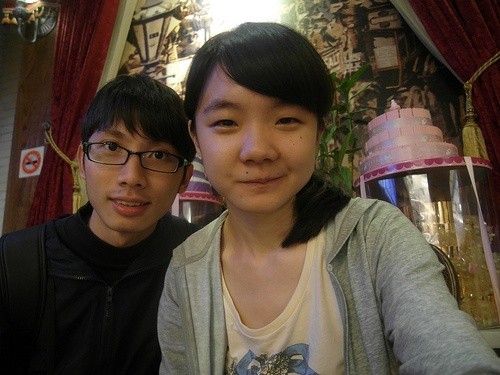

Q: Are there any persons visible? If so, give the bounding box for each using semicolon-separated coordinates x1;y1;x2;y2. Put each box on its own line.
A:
0;72;204;375
157;22;500;375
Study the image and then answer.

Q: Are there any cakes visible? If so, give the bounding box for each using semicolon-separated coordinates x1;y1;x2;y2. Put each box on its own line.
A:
358;107;459;176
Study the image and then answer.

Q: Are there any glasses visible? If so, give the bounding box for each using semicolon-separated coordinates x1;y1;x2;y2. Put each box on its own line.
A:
83;141;188;173
